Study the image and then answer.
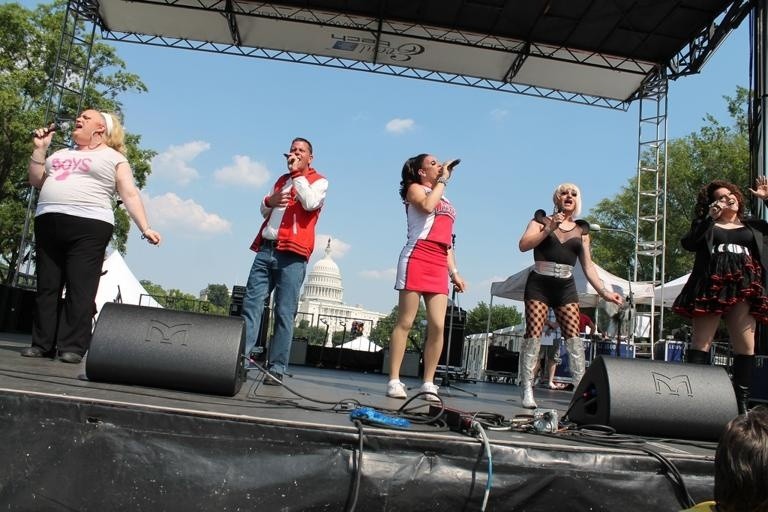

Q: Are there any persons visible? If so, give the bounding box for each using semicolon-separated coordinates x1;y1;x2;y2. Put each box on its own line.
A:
672;175;767;415
20;107;163;364
518;181;625;410
677;402;767;510
239;136;330;385
577;311;596;348
385;152;467;403
532;305;563;390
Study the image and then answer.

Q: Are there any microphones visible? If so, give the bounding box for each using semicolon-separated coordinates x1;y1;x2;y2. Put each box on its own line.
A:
284;154;289;157
447;159;461;170
557;207;566;228
32;122;69;139
709;201;724;214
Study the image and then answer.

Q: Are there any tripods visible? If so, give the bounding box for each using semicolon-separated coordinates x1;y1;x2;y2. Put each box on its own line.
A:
247;290;293;378
407;233;479;398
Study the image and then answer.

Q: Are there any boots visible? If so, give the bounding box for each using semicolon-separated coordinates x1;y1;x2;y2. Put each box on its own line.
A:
520;338;541;409
566;337;585;393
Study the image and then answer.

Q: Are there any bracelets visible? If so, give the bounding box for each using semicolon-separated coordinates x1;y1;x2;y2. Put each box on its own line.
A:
437;177;450;186
599;288;608;297
449;268;460;278
29;153;47;167
140;226;152;240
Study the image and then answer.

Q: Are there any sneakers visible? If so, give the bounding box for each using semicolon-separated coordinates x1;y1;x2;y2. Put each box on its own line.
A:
423;384;439;401
387;382;407;398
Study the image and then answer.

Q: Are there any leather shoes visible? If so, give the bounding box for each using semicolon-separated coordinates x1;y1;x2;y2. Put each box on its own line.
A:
60;352;81;363
21;348;55;357
263;372;282;385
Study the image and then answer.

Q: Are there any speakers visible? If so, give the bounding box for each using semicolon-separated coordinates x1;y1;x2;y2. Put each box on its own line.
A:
426;325;464;368
86;301;247;397
269;334;308;366
568;356;739;443
487;351;518;374
381;347;421;378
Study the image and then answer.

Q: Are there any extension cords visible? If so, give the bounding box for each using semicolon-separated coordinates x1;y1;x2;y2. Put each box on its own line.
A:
534;408;558;433
